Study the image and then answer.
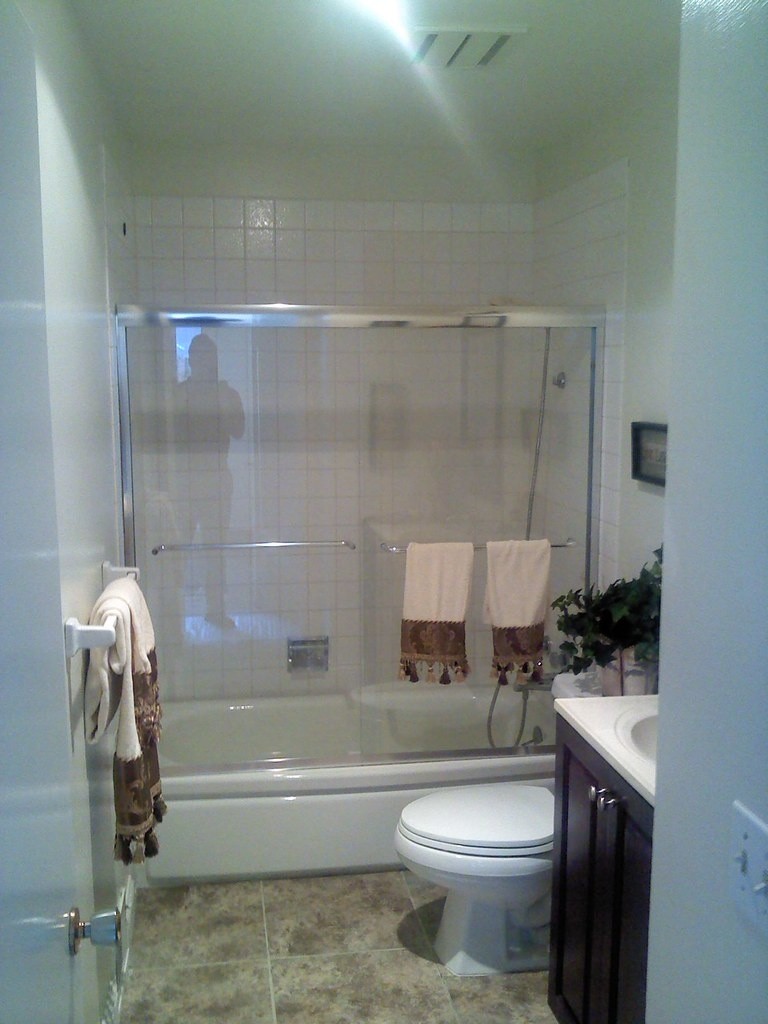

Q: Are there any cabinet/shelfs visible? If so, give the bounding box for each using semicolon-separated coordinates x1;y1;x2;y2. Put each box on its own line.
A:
544;713;651;1022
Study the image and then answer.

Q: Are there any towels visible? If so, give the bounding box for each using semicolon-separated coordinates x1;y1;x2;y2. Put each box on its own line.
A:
83;575;168;868
480;537;552;688
397;540;472;685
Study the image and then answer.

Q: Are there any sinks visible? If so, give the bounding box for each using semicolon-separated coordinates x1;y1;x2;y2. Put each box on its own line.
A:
613;700;659;769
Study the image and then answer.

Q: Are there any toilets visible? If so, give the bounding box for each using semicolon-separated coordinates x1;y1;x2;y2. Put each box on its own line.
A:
392;672;606;978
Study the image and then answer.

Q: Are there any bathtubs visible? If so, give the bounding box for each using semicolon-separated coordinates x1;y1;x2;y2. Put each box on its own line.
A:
89;675;557;879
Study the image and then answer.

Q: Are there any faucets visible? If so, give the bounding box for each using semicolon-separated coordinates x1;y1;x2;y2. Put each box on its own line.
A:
531;656;546;685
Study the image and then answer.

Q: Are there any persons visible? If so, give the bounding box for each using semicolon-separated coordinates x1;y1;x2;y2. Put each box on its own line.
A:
169;333;244;642
